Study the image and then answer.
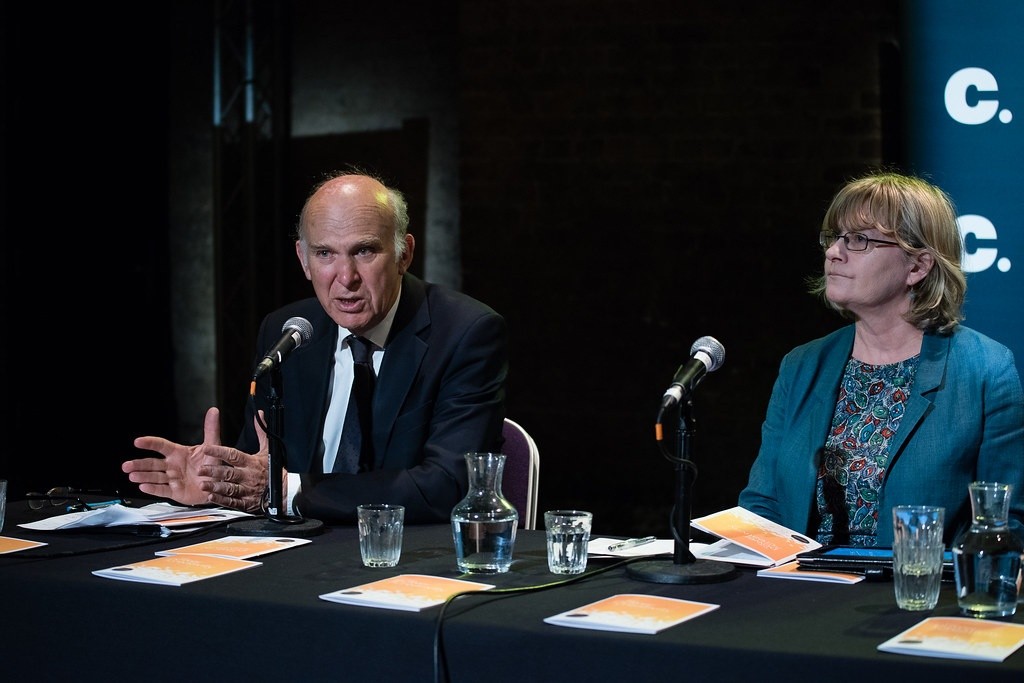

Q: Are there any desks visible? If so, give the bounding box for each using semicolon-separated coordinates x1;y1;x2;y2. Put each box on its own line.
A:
0;494;1024;683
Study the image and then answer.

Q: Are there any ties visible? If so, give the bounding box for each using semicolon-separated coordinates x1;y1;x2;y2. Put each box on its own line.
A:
332;335;378;473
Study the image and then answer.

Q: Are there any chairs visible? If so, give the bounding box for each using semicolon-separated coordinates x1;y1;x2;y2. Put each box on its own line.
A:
500;417;540;530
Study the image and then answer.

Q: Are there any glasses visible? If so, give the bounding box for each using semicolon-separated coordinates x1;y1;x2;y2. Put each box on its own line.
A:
26;486;126;510
818;229;923;251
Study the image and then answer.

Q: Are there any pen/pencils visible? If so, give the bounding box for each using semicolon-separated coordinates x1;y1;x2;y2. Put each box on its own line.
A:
66;499;125;512
608;536;657;550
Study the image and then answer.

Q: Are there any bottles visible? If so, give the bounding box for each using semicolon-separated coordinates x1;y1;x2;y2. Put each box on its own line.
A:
952;482;1024;618
451;452;519;575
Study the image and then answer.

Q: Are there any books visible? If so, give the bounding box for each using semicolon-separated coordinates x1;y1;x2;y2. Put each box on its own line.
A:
543;594;720;634
91;552;263;586
878;616;1024;663
138;514;226;527
154;536;312;560
0;535;49;555
690;506;866;583
318;574;496;612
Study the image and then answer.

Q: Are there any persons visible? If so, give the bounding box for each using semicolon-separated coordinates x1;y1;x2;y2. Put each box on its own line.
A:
735;173;1023;550
122;174;509;525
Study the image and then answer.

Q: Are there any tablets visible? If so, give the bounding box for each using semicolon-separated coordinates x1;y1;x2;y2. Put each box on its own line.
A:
796;544;955;585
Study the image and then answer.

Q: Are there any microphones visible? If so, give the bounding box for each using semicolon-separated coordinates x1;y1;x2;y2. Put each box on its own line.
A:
661;335;725;409
253;317;314;378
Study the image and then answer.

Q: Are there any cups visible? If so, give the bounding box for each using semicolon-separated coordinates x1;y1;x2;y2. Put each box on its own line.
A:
0;482;8;532
892;545;945;611
358;504;405;568
893;506;945;547
544;511;592;575
